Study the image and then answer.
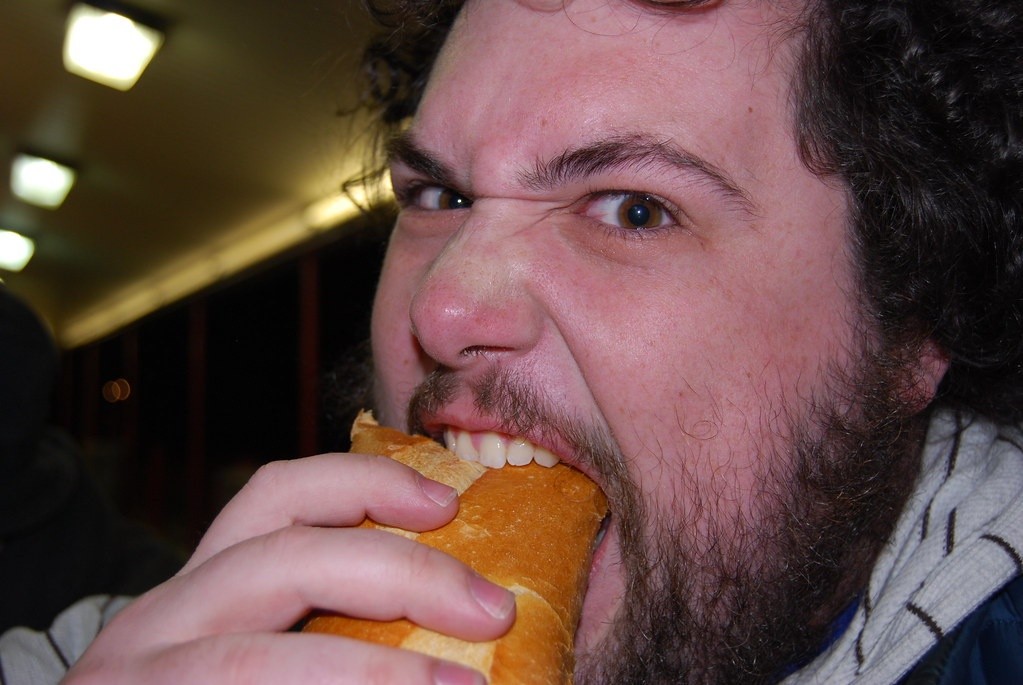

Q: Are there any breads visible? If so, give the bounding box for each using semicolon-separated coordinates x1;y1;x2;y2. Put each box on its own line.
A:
290;408;610;685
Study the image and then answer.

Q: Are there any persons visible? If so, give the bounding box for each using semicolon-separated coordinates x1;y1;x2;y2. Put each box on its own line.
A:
1;0;1023;685
